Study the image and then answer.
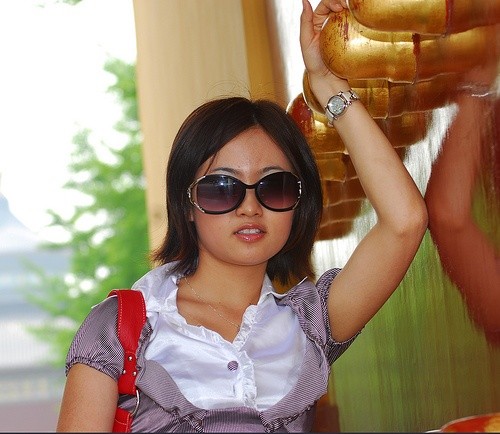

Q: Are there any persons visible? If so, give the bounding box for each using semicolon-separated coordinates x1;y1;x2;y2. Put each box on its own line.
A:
55;0;427;430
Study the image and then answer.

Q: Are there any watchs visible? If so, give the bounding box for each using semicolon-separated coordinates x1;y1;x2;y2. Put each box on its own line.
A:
324;88;361;128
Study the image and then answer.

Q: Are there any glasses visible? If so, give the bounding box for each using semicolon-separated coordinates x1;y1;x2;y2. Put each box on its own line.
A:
185;169;304;215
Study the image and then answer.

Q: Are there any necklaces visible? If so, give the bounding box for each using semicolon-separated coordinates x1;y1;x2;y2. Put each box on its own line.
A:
184;275;241;333
